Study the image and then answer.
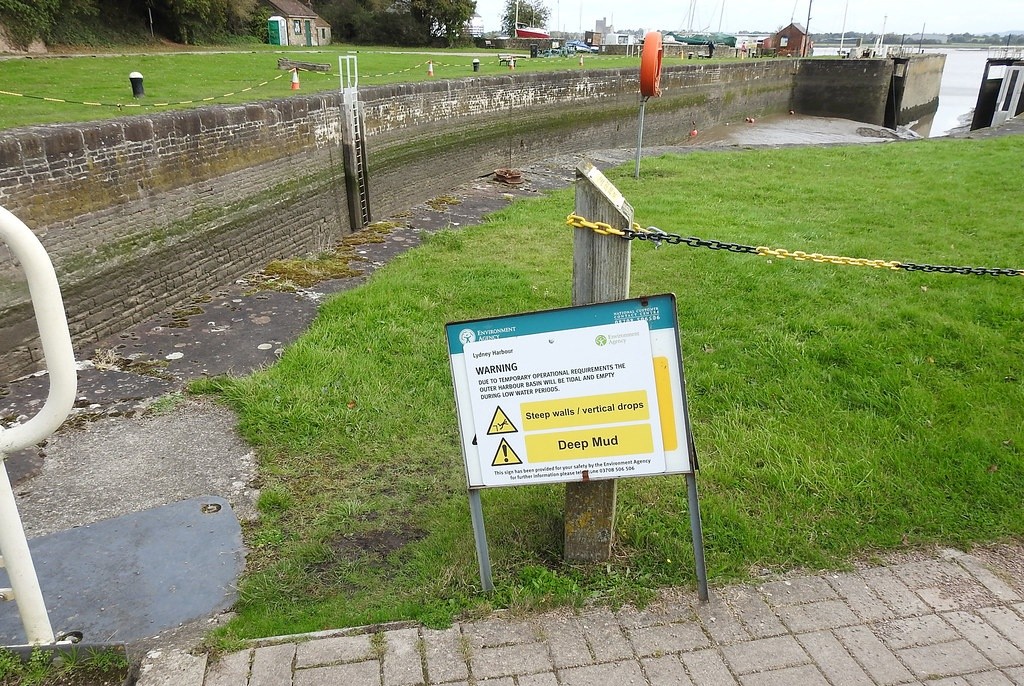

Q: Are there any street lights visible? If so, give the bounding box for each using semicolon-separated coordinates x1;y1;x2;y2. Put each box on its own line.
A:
879;16;887;56
557;0;560;38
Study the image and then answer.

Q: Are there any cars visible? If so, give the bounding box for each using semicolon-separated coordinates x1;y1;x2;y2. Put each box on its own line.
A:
589;47;600;53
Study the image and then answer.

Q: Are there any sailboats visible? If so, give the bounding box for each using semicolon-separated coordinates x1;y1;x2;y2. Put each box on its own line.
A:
675;0;737;47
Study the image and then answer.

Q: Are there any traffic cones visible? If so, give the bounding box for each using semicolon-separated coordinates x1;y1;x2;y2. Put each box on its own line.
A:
509;56;515;69
291;66;300;90
579;55;584;66
427;60;434;76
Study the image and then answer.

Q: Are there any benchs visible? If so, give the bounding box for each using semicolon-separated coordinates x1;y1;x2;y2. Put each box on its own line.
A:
499;53;517;68
697;50;710;59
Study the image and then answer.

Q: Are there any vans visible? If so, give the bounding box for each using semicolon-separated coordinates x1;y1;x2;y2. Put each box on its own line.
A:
757;40;765;47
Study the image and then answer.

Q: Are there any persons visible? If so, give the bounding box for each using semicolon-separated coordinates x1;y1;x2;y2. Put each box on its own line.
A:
742;42;746;52
708;42;715;56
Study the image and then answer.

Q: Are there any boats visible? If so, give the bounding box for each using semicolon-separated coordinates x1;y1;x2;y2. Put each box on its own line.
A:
516;27;551;38
566;39;591;52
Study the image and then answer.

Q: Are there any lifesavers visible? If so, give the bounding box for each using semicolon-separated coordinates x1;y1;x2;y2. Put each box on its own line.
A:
640;32;664;98
